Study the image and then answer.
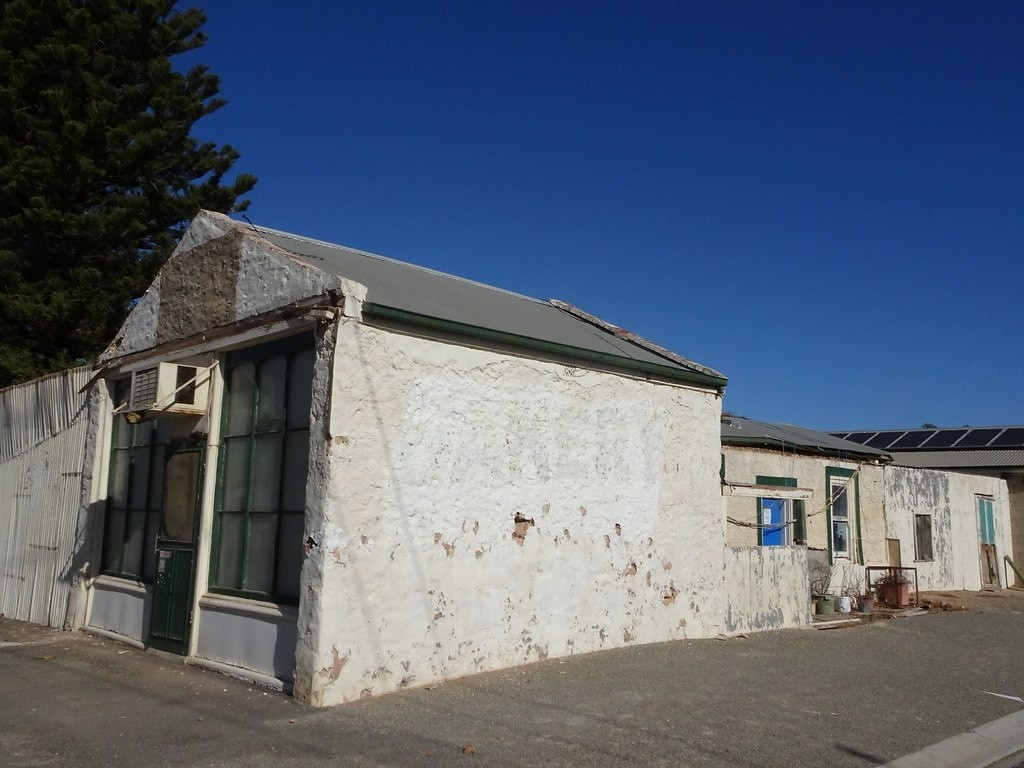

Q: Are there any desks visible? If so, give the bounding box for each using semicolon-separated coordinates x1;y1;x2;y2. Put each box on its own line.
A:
867;567;919;609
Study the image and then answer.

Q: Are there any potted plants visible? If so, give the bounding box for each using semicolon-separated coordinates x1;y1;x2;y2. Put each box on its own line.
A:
874;573;913;606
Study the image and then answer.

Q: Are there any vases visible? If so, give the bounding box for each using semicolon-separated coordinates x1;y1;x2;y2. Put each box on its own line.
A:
835;597;851;612
811;594;834;615
857;596;875;612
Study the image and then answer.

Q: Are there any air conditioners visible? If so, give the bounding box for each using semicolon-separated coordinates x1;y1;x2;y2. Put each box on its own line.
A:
129;362;210;419
